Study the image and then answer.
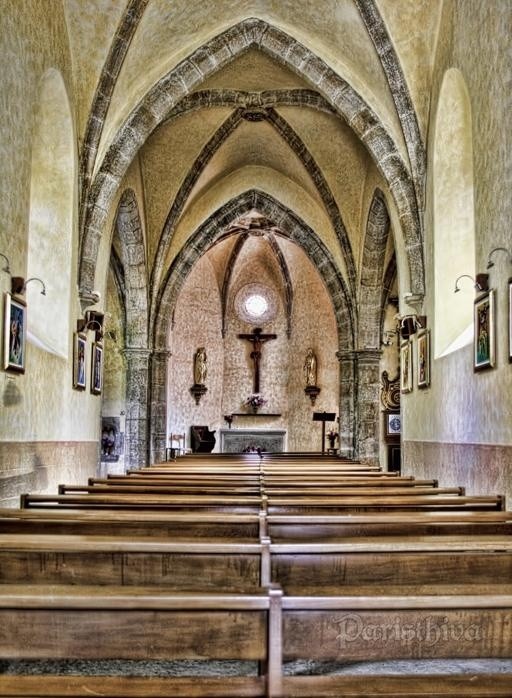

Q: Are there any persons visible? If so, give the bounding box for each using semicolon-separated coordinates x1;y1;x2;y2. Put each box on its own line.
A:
194;346;206;385
244;334;273;370
104;429;115;454
303;347;318;385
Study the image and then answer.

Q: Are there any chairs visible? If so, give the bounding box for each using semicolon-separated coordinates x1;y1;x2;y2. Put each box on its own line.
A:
170;432;193;456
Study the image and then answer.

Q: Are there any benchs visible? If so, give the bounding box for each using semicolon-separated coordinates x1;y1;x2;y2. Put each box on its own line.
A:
0;448;512;698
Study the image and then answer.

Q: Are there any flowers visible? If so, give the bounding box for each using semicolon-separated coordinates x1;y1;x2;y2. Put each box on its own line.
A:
245;394;267;409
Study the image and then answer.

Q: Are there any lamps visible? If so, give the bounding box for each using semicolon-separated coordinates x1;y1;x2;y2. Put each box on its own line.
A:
12;276;47;296
485;248;511;270
77;319;103;335
0;252;12;274
400;314;427;329
454;273;488;293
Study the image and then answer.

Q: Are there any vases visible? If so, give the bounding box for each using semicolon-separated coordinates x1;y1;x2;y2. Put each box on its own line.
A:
252;406;259;413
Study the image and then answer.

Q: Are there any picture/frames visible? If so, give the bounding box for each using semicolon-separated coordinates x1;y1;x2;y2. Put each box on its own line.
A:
72;329;104;396
473;287;498;374
400;327;431;394
5;291;28;372
